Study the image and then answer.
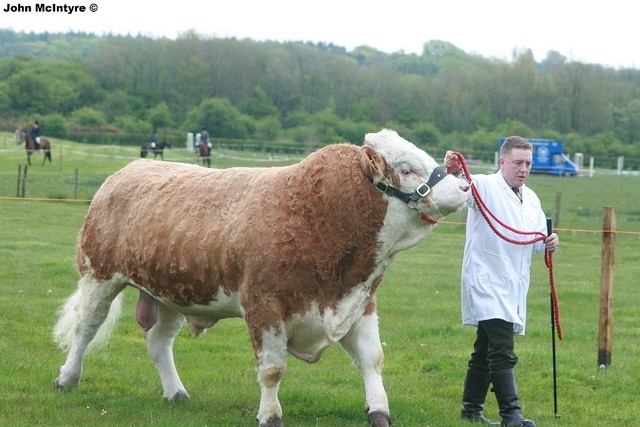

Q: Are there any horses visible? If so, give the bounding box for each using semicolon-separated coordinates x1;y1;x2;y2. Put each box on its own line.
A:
195;131;211;169
15;127;52;166
140;139;171;160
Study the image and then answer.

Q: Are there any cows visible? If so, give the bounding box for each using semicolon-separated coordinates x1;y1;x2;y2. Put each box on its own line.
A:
51;128;473;427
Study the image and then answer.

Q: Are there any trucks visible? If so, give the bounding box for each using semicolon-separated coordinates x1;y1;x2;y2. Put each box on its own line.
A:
493;136;579;177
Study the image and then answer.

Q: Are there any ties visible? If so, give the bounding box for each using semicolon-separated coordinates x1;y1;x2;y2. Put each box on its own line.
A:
513;186;517;194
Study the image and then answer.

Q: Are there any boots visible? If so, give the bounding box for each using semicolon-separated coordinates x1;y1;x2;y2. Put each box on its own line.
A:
462;369;491;424
491;370;535;426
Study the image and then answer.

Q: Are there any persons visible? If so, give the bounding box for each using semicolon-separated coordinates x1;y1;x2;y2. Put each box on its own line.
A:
444;136;561;421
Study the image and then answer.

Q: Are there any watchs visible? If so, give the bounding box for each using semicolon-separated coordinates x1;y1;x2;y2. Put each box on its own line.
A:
451;170;460;175
